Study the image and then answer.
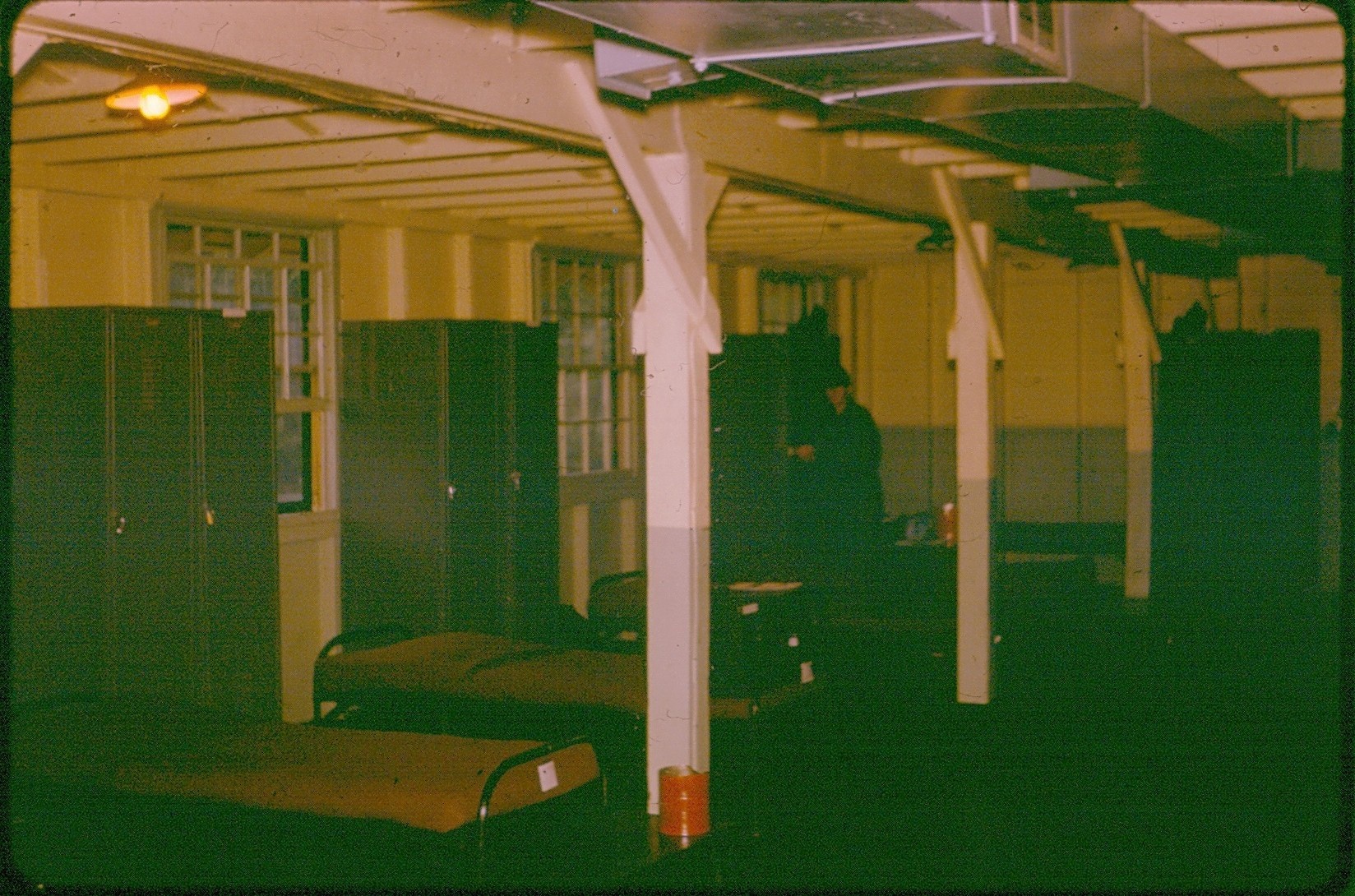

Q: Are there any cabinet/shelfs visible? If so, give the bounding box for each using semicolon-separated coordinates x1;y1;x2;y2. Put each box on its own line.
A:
1151;329;1321;592
343;319;559;651
9;306;281;719
711;331;840;550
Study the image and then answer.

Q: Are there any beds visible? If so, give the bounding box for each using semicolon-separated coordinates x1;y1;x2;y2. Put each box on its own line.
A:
8;550;1094;885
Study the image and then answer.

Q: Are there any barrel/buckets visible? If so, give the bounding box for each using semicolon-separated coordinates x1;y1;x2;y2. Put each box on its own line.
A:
659;766;710;837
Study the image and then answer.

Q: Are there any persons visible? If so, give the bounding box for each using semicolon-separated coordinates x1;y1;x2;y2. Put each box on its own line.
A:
755;358;887;525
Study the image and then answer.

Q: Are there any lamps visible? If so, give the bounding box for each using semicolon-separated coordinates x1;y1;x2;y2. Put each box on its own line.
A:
106;69;207;120
